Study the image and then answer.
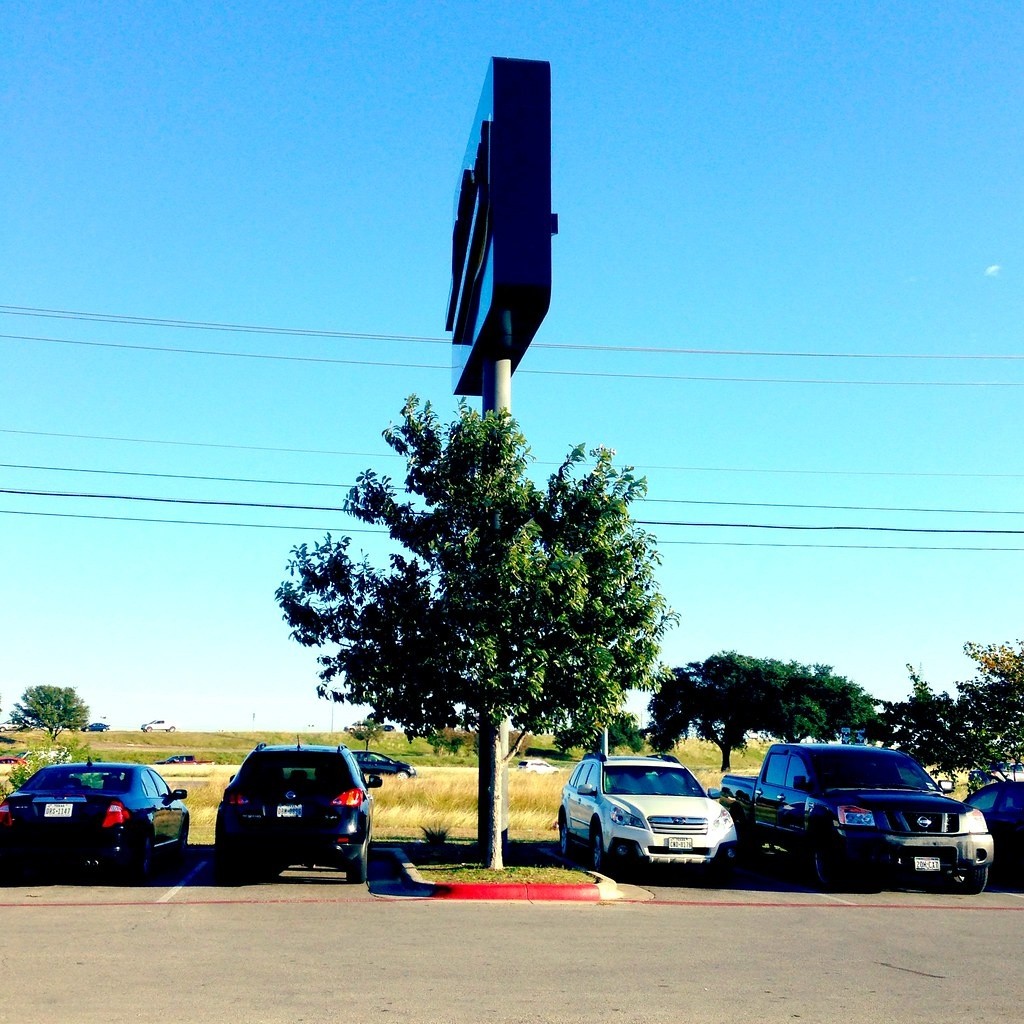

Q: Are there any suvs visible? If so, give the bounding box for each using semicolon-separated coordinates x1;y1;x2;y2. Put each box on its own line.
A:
214;742;383;885
558;753;737;874
517;759;560;775
0;718;26;732
141;718;178;733
344;720;375;734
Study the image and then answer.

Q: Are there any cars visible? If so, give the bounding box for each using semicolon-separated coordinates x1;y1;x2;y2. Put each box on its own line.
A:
0;756;27;764
80;723;110;733
350;751;416;780
377;725;396;732
960;780;1024;883
0;762;190;878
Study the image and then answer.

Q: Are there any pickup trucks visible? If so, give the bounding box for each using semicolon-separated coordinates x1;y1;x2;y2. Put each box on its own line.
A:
719;742;995;894
155;755;214;766
968;762;1024;785
